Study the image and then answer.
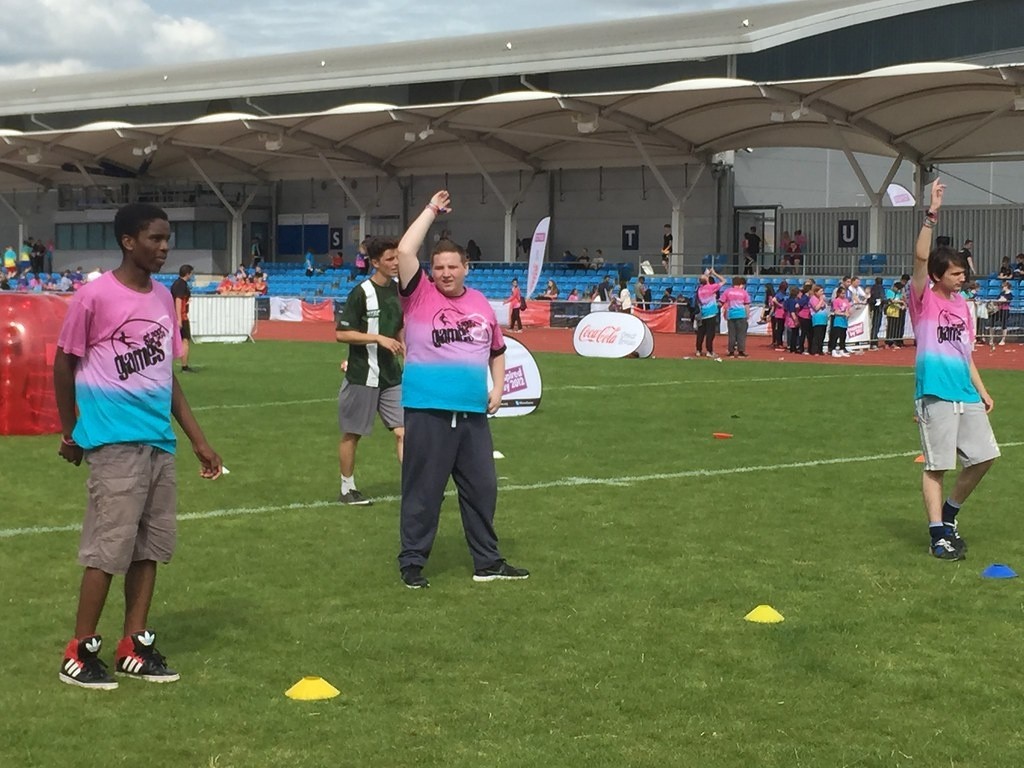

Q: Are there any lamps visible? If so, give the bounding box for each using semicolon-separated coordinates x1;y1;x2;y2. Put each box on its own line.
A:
419;123;433;140
1010;85;1024;111
404;125;415;142
132;141;156;156
27;148;42;163
265;131;283;151
571;112;601;133
771;101;809;123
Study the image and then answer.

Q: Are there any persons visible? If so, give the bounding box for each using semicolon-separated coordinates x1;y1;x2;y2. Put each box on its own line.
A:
536;225;868;357
170;265;196;374
909;176;1001;563
54;201;222;690
868;236;1024;352
441;228;450;238
0;236;103;292
303;247;316;277
396;190;531;588
357;234;377;275
502;277;524;334
334;235;406;505
324;251;344;268
249;237;263;268
466;240;482;261
216;264;268;297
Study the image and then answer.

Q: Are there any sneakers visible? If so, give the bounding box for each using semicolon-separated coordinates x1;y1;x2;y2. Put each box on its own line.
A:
114;631;180;684
928;537;959;561
400;561;431;589
942;520;968;554
58;638;119;691
472;560;530;581
337;487;373;506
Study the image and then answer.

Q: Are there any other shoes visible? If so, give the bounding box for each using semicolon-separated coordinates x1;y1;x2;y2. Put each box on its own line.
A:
998;340;1006;345
727;352;735;358
706;350;720;357
696;350;702;357
738;352;750;358
747;259;755;266
504;327;514;333
977;340;985;344
182;366;196;373
514;329;523;334
770;341;906;358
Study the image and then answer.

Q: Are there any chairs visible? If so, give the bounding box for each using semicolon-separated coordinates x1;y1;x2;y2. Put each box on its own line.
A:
154;253;1024;312
0;261;86;292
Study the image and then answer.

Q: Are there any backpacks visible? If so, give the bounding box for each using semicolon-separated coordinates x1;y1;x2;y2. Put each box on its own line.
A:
520;296;526;311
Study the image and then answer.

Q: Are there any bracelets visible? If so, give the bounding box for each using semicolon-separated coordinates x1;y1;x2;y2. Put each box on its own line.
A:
62;435;78;445
922;209;937;228
425;202;440;219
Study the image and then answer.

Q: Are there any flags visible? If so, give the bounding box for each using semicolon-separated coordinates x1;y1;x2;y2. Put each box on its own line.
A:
526;216;551;297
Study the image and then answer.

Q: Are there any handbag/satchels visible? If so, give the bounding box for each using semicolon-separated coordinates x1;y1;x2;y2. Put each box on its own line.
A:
886;305;899;317
